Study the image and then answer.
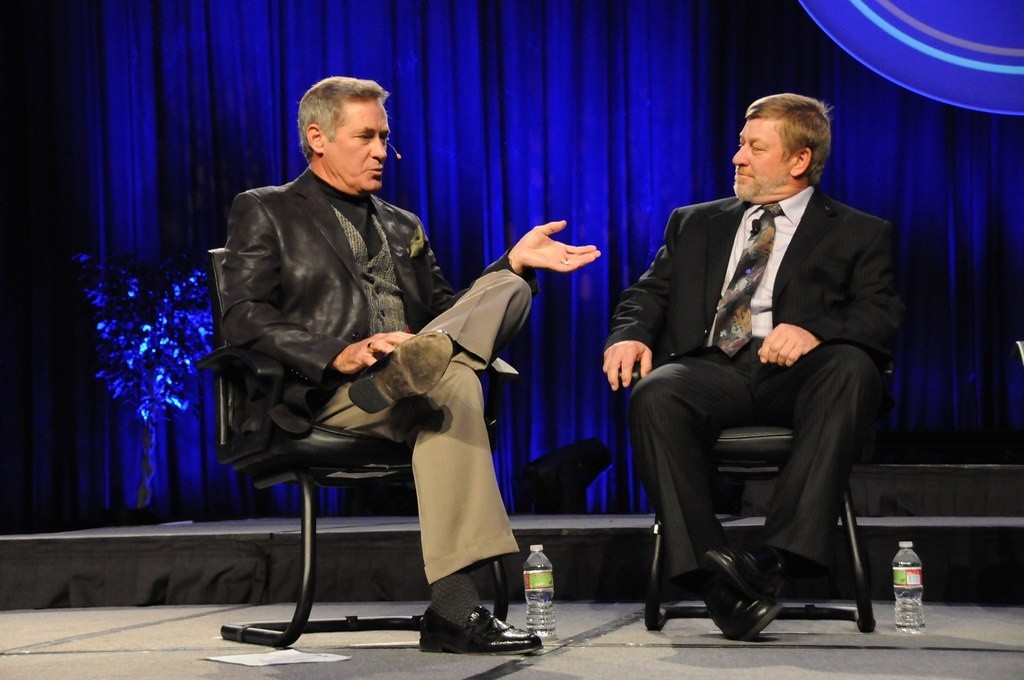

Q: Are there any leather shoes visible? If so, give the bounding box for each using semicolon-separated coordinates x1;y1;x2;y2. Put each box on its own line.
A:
348;325;454;416
418;603;543;656
706;595;785;642
705;547;779;599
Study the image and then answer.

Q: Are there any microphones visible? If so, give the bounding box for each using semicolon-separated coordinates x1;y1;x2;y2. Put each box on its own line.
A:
387;142;401;159
752;219;761;233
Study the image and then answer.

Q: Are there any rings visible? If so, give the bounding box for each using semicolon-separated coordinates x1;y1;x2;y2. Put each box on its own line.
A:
368;342;374;353
565;258;569;265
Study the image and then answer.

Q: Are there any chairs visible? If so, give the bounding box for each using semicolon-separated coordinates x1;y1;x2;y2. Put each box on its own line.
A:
618;355;897;633
193;246;519;646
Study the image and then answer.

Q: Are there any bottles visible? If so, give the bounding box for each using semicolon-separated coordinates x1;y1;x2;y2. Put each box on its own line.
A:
891;542;925;634
523;544;557;637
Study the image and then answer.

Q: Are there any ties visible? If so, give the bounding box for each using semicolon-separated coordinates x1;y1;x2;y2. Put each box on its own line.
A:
713;204;785;360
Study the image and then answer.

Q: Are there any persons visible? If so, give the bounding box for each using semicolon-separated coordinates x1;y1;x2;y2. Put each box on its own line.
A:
604;93;901;640
220;77;600;654
